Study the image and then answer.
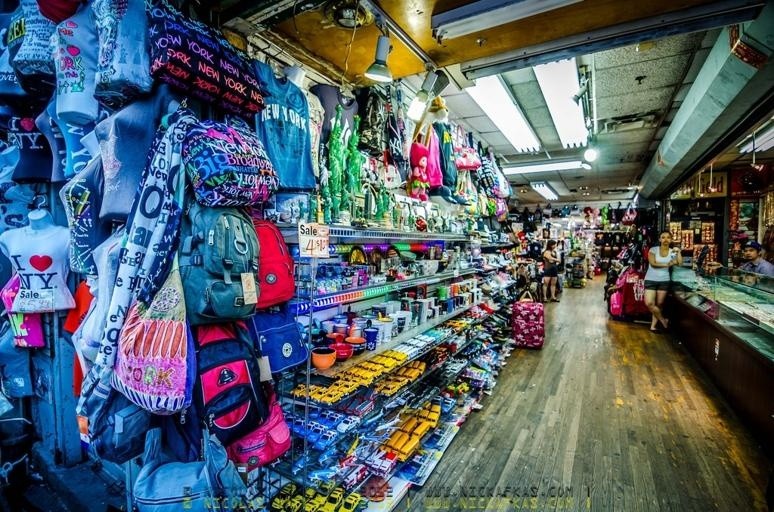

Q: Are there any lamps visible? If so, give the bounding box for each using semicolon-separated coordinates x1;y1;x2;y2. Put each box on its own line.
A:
571;84;588;106
364;35;392;84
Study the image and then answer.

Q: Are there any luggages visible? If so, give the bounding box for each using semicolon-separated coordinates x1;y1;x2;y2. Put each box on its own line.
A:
510;301;546;350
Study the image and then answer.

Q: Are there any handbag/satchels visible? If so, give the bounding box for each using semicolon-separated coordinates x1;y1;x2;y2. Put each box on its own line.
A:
143;0;267;120
450;124;512;224
521;200;650;316
173;105;280;211
247;311;311;373
132;423;256;511
226;385;297;474
104;296;194;416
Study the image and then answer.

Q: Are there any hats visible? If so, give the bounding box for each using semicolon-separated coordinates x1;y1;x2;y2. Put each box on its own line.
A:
740;240;762;251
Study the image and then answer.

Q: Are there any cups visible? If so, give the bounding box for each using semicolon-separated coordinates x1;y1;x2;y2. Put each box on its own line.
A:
320;298;432;350
427;279;481;320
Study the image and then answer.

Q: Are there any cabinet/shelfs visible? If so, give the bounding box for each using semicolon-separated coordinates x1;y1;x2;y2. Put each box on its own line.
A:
670;260;774;461
241;198;520;511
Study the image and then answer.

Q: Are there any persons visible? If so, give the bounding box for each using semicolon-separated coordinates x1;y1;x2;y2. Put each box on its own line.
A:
542;240;560;302
272;309;499;512
707;241;774;275
644;230;682;334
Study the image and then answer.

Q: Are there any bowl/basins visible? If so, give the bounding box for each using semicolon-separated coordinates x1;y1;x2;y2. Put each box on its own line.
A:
299;327;367;371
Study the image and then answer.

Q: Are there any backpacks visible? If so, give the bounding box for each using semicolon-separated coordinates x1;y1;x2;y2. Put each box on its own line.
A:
74;362;159;463
174;200;261;326
187;323;270;451
246;217;296;310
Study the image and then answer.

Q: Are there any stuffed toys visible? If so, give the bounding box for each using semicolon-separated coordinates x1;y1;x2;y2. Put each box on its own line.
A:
438;186;469;205
408;144;430;201
